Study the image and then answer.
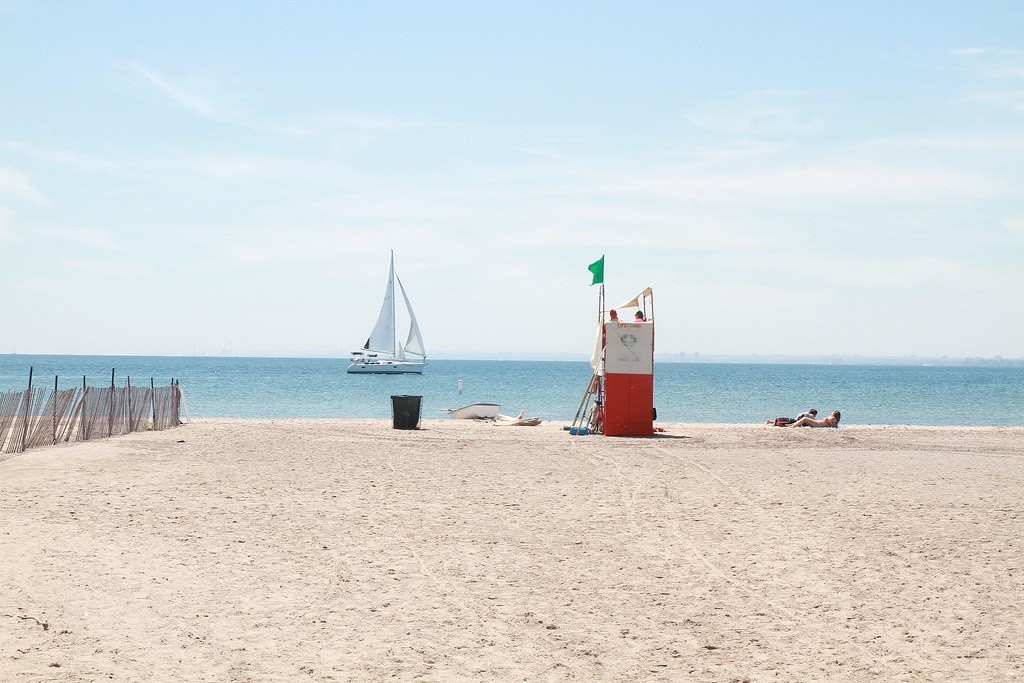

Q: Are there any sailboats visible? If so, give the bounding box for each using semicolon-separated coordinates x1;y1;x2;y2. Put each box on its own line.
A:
346;250;429;376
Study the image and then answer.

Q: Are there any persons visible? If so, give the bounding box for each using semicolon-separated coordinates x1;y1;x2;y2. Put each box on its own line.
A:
633;311;646;323
608;310;624;323
784;409;841;428
796;408;818;421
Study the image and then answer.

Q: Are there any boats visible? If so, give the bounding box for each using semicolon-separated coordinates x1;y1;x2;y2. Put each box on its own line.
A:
446;401;500;421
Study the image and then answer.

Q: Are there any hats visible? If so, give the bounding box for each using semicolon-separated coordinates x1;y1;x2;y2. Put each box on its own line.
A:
610;309;616;314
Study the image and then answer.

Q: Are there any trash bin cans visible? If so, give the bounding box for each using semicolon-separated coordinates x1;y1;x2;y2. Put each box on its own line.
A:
390;394;423;428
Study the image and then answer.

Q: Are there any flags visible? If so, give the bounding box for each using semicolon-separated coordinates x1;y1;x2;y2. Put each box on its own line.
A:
588;254;604;287
